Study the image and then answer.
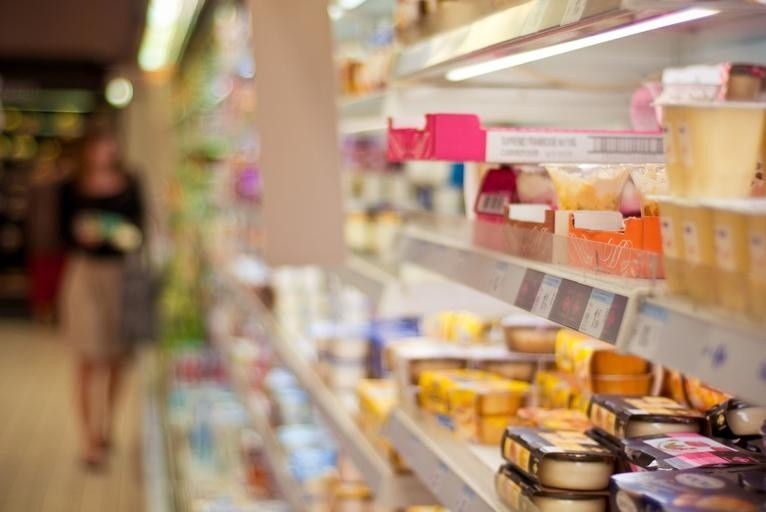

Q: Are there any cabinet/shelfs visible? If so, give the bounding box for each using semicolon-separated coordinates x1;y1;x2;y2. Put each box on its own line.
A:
137;0;765;512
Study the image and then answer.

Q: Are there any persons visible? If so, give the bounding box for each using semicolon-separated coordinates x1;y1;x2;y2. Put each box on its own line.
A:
58;124;153;474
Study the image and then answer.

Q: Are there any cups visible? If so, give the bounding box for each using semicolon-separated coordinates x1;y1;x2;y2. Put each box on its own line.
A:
539;97;766;319
